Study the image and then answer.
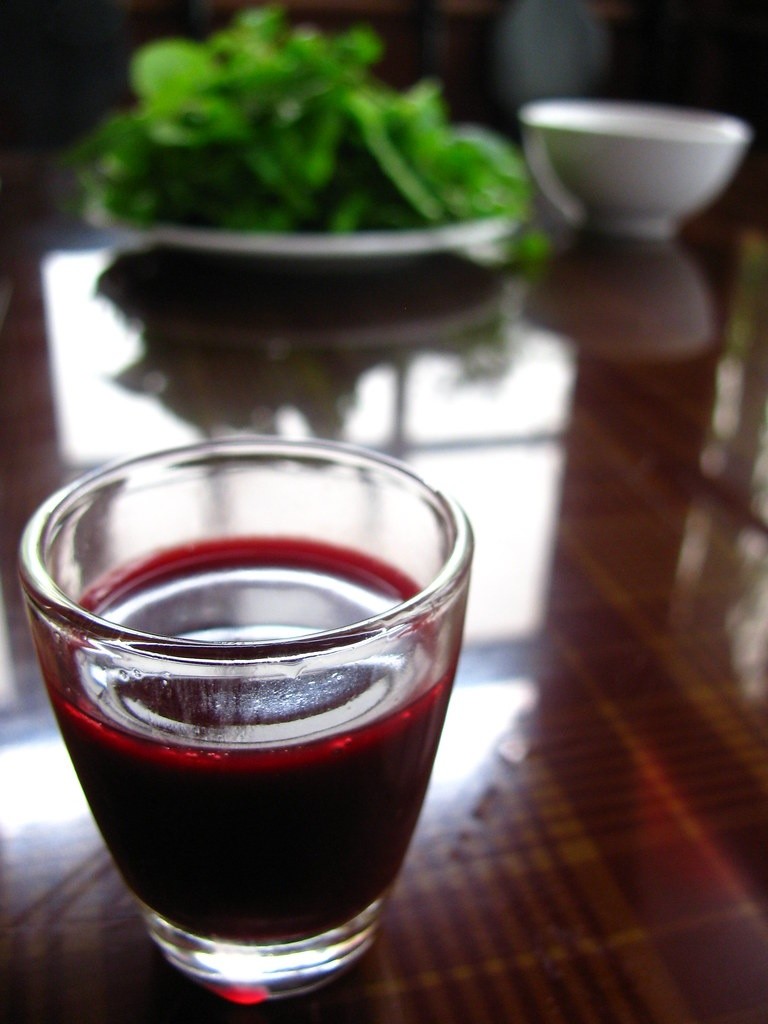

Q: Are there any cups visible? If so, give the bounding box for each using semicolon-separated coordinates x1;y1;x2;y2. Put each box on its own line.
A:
16;435;473;1001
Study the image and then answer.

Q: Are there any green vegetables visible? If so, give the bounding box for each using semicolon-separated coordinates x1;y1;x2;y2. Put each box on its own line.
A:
70;2;554;285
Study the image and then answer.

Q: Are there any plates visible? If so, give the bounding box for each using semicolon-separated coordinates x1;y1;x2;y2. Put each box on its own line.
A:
90;205;510;258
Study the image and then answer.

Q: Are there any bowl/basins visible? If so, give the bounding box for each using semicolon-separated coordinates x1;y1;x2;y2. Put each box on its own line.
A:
516;99;753;247
